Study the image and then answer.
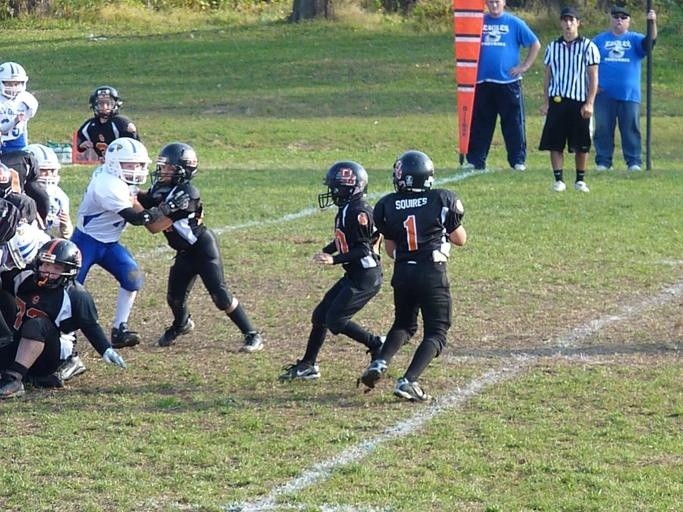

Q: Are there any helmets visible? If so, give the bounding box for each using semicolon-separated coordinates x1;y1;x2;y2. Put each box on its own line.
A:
393;151;435;191
318;161;370;208
106;138;153;185
153;142;199;185
88;86;123;118
0;62;28;98
0;142;82;289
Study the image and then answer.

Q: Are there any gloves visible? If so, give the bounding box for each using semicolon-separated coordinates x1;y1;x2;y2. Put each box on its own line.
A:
161;185;190;214
102;347;128;372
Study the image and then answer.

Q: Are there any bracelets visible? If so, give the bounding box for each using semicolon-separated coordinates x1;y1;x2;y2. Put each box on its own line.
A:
524;62;530;69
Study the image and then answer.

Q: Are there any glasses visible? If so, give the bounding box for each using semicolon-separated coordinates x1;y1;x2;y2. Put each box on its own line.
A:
614;14;627;19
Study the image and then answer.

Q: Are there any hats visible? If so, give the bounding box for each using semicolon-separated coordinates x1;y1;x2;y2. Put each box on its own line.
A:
611;5;630;15
561;7;579;20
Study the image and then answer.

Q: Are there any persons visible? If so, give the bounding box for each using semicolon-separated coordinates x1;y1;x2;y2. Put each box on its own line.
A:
126;141;265;353
589;3;659;171
534;7;604;193
1;144;126;398
68;137;153;349
276;159;388;382
463;0;541;173
77;86;138;160
1;62;38;152
352;149;469;405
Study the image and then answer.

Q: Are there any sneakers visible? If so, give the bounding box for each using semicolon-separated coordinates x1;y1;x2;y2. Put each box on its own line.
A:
457;163;476;170
514;163;526;171
362;335;432;402
111;321;141;349
279;358;321;380
574;165;642;192
159;316;195;347
240;329;264;353
54;352;87;381
553;180;566;192
0;373;26;399
